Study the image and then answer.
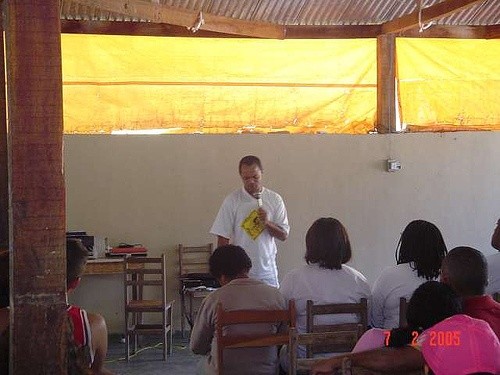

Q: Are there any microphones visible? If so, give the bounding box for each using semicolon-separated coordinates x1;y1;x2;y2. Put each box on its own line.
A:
255;193;263;207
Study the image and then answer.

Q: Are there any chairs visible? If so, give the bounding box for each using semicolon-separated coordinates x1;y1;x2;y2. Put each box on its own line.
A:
289;324;364;375
215;298;297;375
178;242;213;342
123;253;176;362
305;298;368;359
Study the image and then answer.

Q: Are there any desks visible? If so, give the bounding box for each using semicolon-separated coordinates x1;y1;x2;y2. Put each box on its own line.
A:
80;259;144;336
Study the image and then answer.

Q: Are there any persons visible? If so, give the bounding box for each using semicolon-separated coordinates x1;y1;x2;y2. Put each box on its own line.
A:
189;244;290;375
0;238;111;375
279;216;500;375
210;156;289;291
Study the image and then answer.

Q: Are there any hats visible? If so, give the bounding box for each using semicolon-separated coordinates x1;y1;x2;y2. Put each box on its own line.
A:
420;314;500;375
